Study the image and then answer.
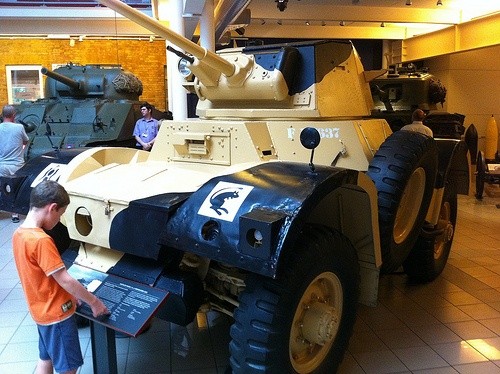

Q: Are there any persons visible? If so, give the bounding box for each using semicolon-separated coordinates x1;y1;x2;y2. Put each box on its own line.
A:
133;103;159;152
401;109;433;140
0;105;29;223
12;180;110;374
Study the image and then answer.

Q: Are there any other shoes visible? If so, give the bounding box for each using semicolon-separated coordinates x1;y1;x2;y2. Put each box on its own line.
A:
11;213;20;223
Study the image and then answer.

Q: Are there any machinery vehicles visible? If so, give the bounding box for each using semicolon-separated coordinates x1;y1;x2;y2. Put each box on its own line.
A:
21;1;474;370
0;60;175;213
